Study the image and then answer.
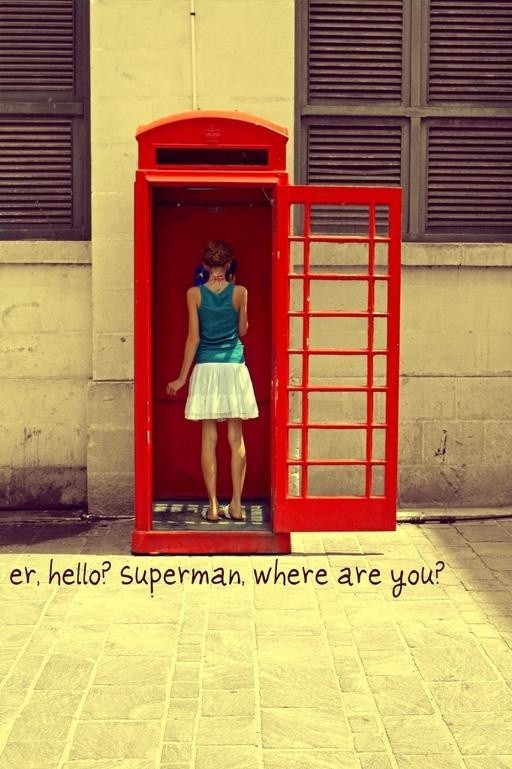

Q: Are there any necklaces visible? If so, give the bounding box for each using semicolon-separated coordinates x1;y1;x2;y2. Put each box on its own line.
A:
210;273;225;283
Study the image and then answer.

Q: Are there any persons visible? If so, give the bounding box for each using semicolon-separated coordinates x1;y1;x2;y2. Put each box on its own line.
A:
165;236;262;524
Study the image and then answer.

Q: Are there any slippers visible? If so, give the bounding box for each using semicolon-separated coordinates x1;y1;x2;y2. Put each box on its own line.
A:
220;505;244;522
201;509;219;523
